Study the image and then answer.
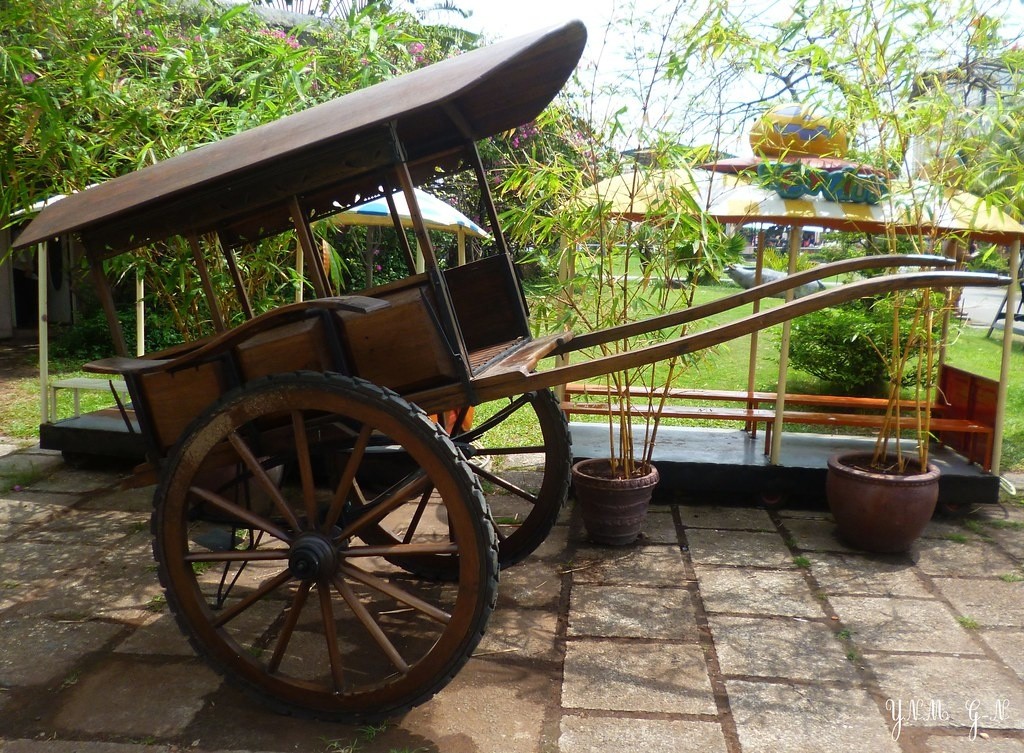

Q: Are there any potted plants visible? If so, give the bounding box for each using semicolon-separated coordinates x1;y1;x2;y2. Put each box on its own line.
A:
479;0;1023;556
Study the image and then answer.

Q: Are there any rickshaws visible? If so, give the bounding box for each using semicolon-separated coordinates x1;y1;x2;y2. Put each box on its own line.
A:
13;15;1016;725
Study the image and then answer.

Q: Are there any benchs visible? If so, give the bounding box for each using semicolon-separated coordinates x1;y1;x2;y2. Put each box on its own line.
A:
566;382;947;448
50;376;129;422
556;398;996;471
